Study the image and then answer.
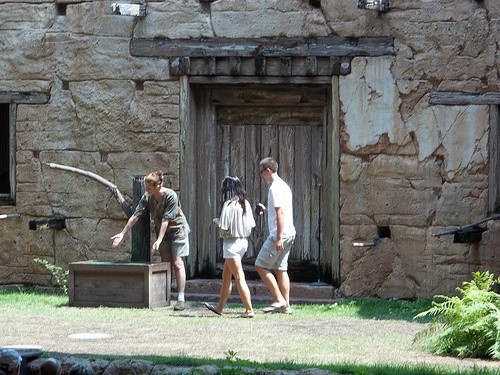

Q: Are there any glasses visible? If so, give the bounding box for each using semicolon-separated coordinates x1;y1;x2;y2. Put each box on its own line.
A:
259;168;268;174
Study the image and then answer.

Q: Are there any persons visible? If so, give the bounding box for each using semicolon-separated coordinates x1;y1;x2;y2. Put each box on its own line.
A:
110;169;190;311
0;348;95;375
205;176;256;318
254;157;296;314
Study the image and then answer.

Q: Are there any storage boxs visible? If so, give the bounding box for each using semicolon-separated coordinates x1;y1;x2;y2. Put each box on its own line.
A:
67;261;171;310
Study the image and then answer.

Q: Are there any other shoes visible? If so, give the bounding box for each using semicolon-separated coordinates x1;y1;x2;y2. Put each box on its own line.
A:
174;300;186;310
262;303;288;312
273;307;291;314
241;309;254;318
204;300;223;315
